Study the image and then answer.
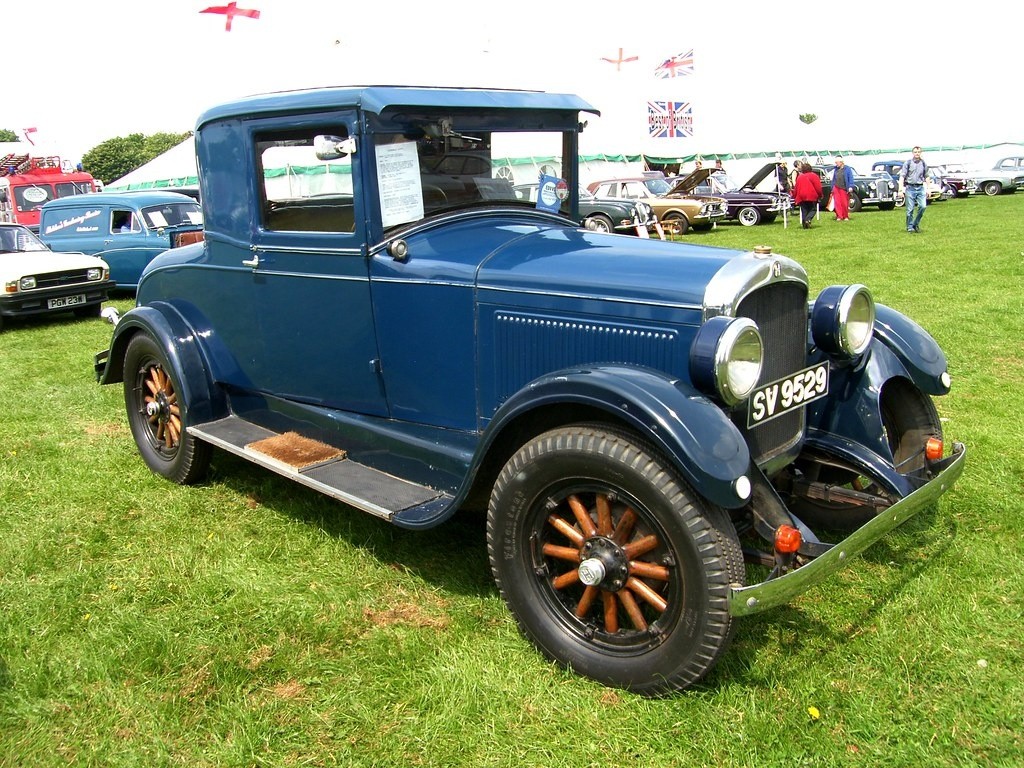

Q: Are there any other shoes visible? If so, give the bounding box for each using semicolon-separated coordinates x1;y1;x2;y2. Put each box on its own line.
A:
907;225;920;233
837;217;849;222
804;221;811;229
790;214;799;217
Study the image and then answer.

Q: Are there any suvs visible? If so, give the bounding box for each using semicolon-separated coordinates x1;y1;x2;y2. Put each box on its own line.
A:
94;84;965;700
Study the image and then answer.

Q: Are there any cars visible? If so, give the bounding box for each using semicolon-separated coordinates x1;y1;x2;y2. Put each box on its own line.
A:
0;221;111;331
808;156;1024;210
507;162;793;234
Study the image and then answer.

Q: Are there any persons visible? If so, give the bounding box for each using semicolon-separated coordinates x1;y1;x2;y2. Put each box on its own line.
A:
658;146;932;233
120;213;140;232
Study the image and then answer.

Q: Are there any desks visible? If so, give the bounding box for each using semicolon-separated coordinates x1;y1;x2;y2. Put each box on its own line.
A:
614;219;656;239
660;218;684;241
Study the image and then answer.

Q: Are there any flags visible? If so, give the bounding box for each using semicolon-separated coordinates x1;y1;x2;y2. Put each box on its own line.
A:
598;48;697;140
21;128;39;145
197;0;263;31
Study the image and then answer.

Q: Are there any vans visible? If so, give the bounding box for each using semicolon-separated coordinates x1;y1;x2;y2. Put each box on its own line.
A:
39;189;205;292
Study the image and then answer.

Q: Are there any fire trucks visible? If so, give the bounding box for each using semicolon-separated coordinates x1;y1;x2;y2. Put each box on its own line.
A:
0;153;95;229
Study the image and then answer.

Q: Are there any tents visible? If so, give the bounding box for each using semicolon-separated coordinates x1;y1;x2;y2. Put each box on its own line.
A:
100;135;1024;200
